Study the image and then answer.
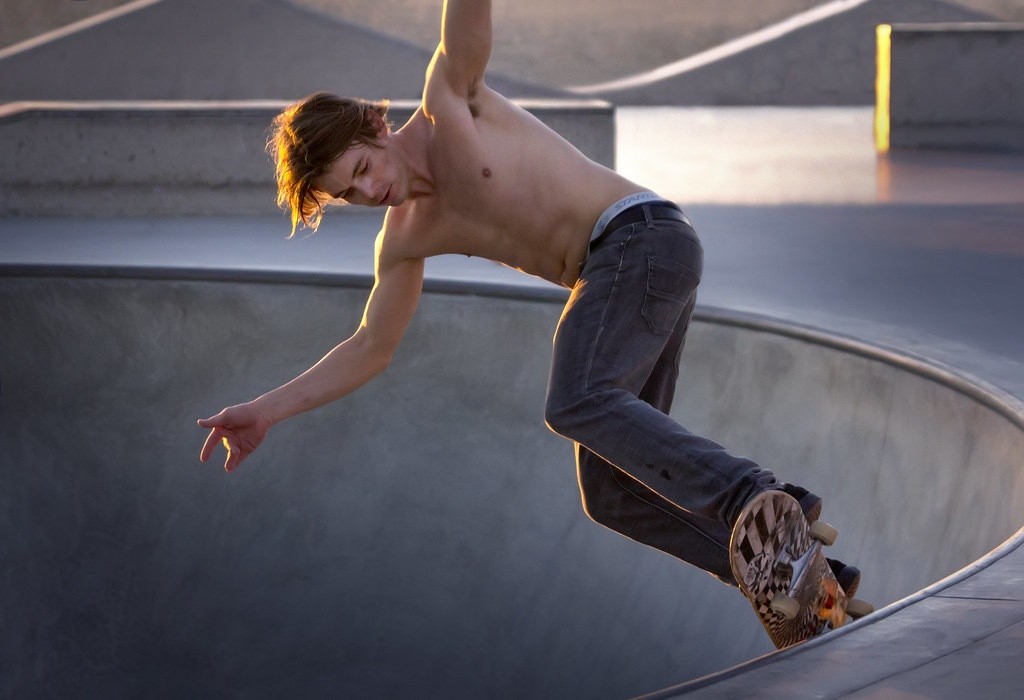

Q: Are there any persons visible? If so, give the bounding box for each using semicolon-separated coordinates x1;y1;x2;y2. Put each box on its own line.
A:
195;1;863;601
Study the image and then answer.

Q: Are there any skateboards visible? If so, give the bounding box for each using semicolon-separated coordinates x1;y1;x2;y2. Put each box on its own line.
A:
728;489;876;652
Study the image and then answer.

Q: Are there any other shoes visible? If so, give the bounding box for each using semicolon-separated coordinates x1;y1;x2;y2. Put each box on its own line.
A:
784;484;822;526
826;558;861;598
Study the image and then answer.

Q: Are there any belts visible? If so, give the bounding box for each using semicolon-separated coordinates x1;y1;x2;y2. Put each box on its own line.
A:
591;206;693;254
